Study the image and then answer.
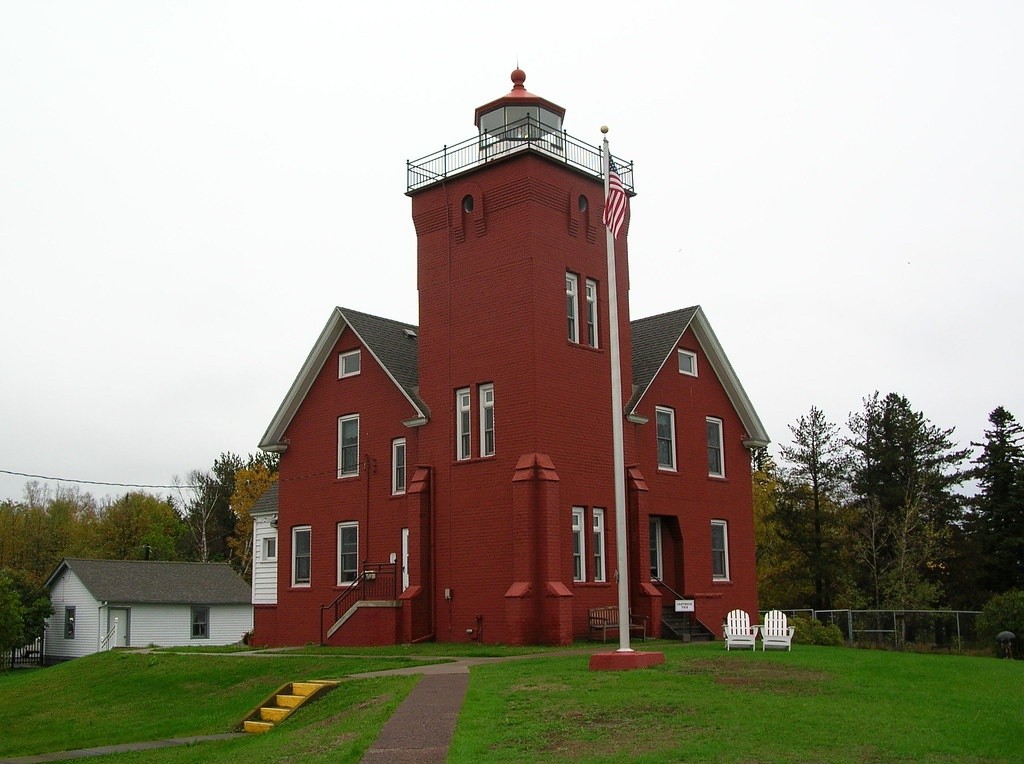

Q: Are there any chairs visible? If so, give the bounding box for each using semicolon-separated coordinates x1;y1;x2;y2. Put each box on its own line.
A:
721;609;758;651
760;610;794;651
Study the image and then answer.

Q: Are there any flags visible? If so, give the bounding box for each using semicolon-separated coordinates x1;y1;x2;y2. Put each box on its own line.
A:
601;149;628;239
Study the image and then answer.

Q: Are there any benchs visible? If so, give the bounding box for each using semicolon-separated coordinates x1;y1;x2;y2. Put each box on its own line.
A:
588;606;646;644
